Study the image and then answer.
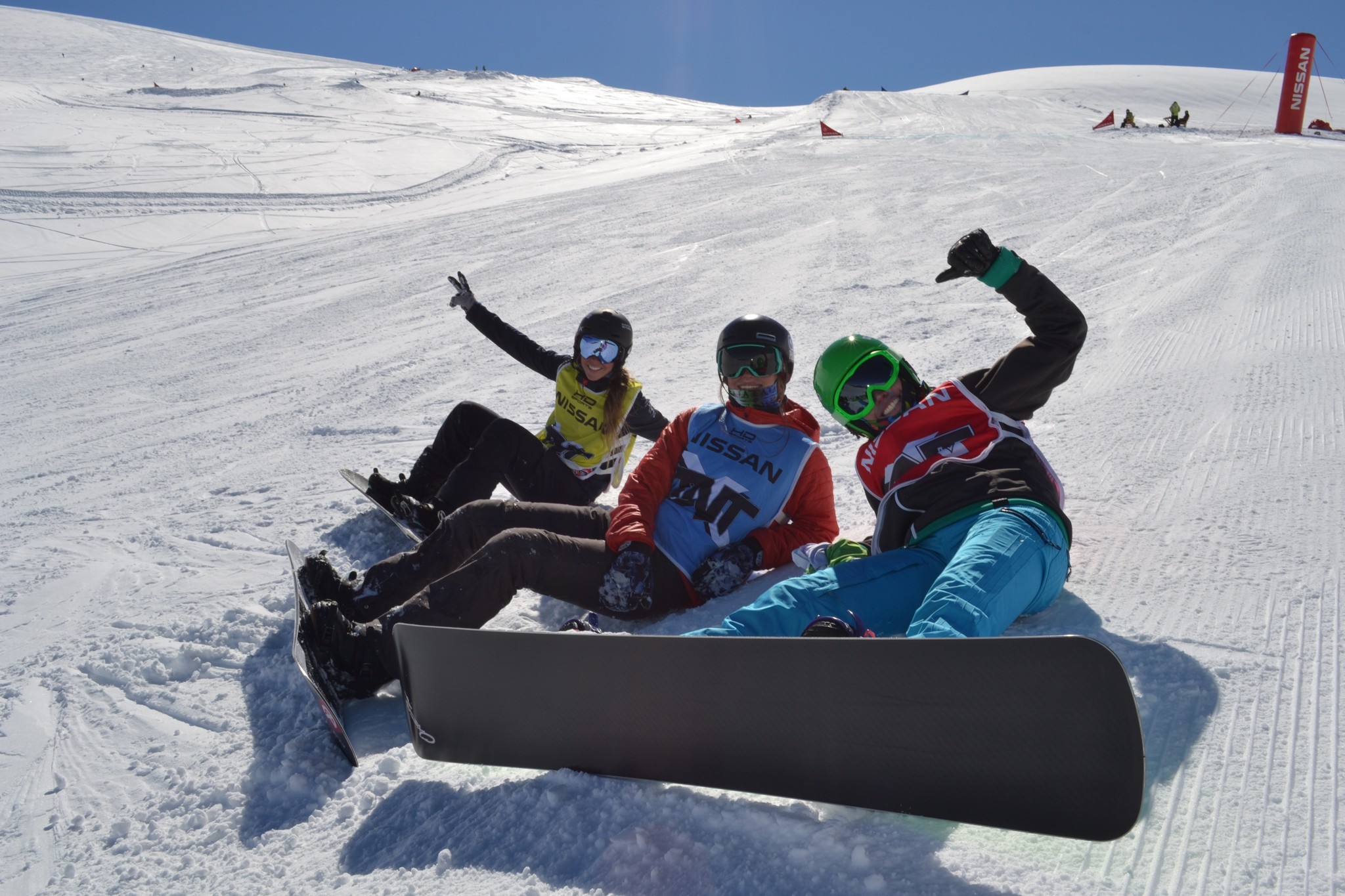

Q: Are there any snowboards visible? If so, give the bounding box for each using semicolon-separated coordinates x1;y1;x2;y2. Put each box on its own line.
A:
338;468;428;543
285;539;361;769
391;622;1145;842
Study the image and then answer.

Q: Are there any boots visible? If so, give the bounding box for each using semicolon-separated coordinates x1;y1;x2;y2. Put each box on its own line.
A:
391;494;455;539
306;550;360;622
311;599;394;700
368;468;415;514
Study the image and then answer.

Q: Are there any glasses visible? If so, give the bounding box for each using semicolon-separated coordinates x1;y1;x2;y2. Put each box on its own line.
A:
831;348;900;422
580;332;618;363
718;344;782;378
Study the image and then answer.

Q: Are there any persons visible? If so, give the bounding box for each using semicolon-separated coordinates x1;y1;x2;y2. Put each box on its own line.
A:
1169;101;1189;128
1120;109;1133;128
560;228;1089;640
369;272;670;537
303;313;840;700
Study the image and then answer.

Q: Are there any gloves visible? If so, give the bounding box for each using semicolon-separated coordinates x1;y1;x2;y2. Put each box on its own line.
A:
447;271;477;315
691;536;764;601
935;228;1001;283
599;540;653;612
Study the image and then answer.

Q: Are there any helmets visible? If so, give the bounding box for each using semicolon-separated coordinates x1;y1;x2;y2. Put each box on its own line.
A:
813;334;921;439
573;308;632;374
716;314;794;383
